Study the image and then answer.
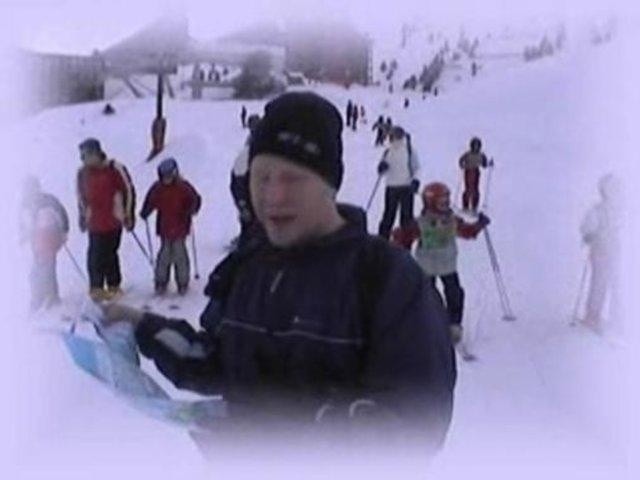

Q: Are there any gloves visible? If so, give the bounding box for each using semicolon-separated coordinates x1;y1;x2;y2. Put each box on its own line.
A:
377;161;388;174
411;179;420;193
479;211;490;226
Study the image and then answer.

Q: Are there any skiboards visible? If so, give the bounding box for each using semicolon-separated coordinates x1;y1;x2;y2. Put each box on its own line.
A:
456;341;474;362
562;308;631;349
143;295;186;310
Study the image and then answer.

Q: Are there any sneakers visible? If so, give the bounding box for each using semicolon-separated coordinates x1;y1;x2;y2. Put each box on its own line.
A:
90;287;119;300
155;281;188;295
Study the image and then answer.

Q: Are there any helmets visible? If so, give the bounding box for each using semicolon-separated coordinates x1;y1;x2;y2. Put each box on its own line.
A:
157;158;178;184
422;182;450;215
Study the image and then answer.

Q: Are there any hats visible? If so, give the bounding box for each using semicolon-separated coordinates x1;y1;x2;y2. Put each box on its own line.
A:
249;91;344;191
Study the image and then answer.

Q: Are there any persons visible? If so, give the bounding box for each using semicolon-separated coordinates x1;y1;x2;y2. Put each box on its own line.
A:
17;177;69;314
77;139;135;305
99;92;456;468
347;99;364;130
139;158;201;296
392;183;491;343
230;115;260;248
581;175;623;334
376;127;421;238
459;138;493;217
242;106;246;127
192;62;219;99
